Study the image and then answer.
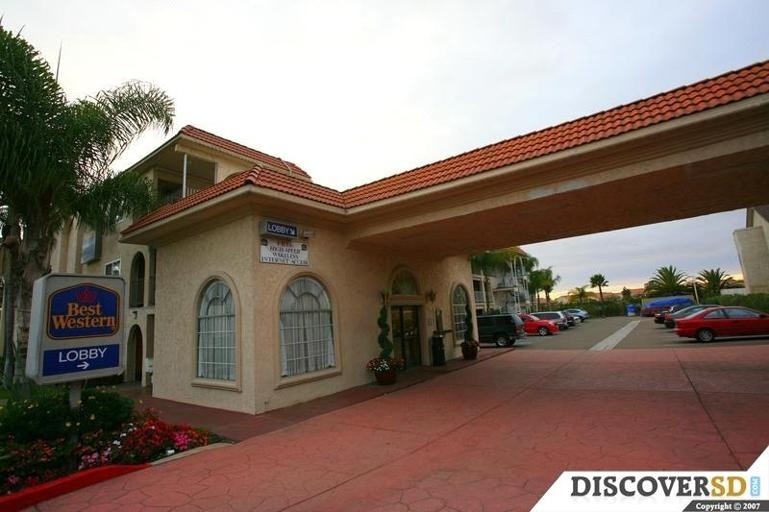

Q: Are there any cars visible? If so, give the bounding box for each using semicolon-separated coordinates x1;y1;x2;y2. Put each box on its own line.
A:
654;303;769;342
519;309;589;336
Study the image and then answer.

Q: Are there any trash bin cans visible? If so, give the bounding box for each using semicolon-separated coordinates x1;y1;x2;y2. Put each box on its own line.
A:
432;336;446;367
627;304;636;317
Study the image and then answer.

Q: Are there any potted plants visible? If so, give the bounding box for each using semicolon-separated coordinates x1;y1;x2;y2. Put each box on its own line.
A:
460;304;479;360
367;306;404;386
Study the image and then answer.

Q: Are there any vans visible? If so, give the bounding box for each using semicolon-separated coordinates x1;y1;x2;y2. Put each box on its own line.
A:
477;314;525;347
